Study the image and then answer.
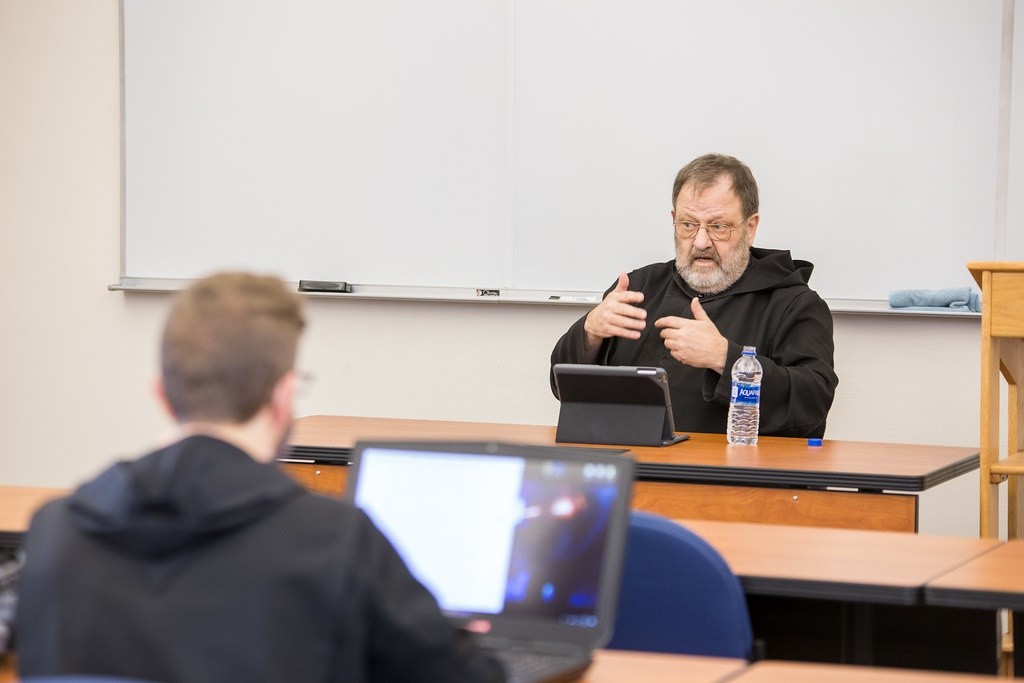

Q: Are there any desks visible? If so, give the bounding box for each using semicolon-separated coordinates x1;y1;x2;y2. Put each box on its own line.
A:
0;484;1003;676
921;537;1024;679
282;414;981;535
963;262;1024;674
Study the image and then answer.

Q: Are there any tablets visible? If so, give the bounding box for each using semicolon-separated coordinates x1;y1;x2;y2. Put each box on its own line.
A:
552;364;676;441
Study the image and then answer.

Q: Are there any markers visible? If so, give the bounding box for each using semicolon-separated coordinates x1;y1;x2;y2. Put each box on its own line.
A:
549;294;597;301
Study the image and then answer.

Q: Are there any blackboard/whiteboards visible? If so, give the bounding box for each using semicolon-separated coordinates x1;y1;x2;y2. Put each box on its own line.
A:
116;0;1015;319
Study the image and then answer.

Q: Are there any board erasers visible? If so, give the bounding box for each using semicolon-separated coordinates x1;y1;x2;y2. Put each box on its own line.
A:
298;279;352;293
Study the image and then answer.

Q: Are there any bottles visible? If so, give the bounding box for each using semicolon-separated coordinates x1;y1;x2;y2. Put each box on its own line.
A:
726;345;763;446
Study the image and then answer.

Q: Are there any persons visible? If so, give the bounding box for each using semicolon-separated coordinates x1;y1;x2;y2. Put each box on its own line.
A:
550;154;839;663
17;270;508;682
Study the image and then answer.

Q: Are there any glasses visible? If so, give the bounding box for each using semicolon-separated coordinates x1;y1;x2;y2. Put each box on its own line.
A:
674;215;749;242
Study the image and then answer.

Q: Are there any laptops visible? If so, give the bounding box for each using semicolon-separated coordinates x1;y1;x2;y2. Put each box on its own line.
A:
342;440;634;683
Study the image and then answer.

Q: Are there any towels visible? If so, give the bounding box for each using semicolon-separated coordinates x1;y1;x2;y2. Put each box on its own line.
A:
889;287;982;312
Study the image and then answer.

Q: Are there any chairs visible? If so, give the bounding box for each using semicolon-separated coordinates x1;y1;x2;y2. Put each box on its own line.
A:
607;511;764;662
